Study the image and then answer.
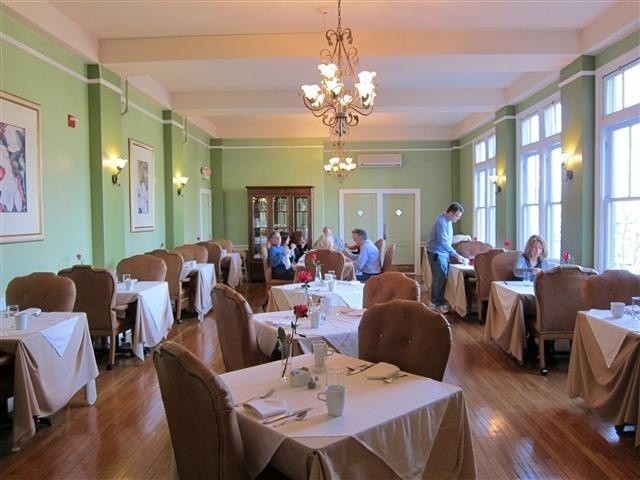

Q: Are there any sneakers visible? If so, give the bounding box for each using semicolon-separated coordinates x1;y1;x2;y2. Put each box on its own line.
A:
429;303;450;315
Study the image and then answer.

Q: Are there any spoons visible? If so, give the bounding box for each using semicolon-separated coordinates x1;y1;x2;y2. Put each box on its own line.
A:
273;410;309;428
345;363;367;370
382;374;408;383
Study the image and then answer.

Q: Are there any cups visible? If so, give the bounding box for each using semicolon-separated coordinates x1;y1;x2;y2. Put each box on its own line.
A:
468;256;475;266
222;249;226;256
611;301;626;319
7;304;19;328
274;223;286;228
631;295;640;322
309;296;332;329
463;258;468;267
317;384;347;418
122;274;130;287
310;340;326;374
325;366;345;390
126;279;134;291
523;271;530;287
323;270;337;291
189;260;196;268
313;344;334;366
14;313;27;331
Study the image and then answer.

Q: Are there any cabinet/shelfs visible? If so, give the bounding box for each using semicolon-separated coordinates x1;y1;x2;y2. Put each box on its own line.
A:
245;184;315;282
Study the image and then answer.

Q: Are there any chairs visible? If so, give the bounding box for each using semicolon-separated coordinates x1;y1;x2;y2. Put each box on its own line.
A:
580;270;640;310
211;283;271;372
118;255;167;344
176;237;247;322
154;341;248;480
58;265;135;371
260;236;421;309
422;235;522;323
363;272;420;309
145;250;193;324
357;299;452;382
6;271;77;312
525;264;597;373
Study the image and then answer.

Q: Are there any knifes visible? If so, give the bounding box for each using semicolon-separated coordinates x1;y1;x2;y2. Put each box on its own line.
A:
347;364;376;376
262;405;314;424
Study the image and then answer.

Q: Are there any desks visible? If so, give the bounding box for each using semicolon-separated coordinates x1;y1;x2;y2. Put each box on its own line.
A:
267;280;366;312
116;280;175;362
567;304;640;434
253;306;364;360
483;280;537;366
2;314;100;454
217;349;475;479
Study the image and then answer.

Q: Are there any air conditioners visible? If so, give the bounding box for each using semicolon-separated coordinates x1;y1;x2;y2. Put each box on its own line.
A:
358;153;402;168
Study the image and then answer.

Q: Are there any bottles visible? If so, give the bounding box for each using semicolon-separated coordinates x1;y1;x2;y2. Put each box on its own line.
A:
255;218;260;227
308;375;320;389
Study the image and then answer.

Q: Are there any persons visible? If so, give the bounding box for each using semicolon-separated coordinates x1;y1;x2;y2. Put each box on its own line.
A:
513;234;559;365
313;226;344;254
344;228;360;254
426;202;468;313
269;231;308;280
342;229;381;283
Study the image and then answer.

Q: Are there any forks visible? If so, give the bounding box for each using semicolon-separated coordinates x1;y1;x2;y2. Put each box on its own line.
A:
235;387;276;407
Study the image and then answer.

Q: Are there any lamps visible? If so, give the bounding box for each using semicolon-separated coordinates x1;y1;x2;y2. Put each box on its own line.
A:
323;138;357;182
176;176;189;196
489;173;502;195
111;158;129;186
555;154;573;181
300;0;378;140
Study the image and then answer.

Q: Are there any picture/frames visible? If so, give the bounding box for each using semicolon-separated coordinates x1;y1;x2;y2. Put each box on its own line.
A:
127;137;155;233
0;91;45;246
